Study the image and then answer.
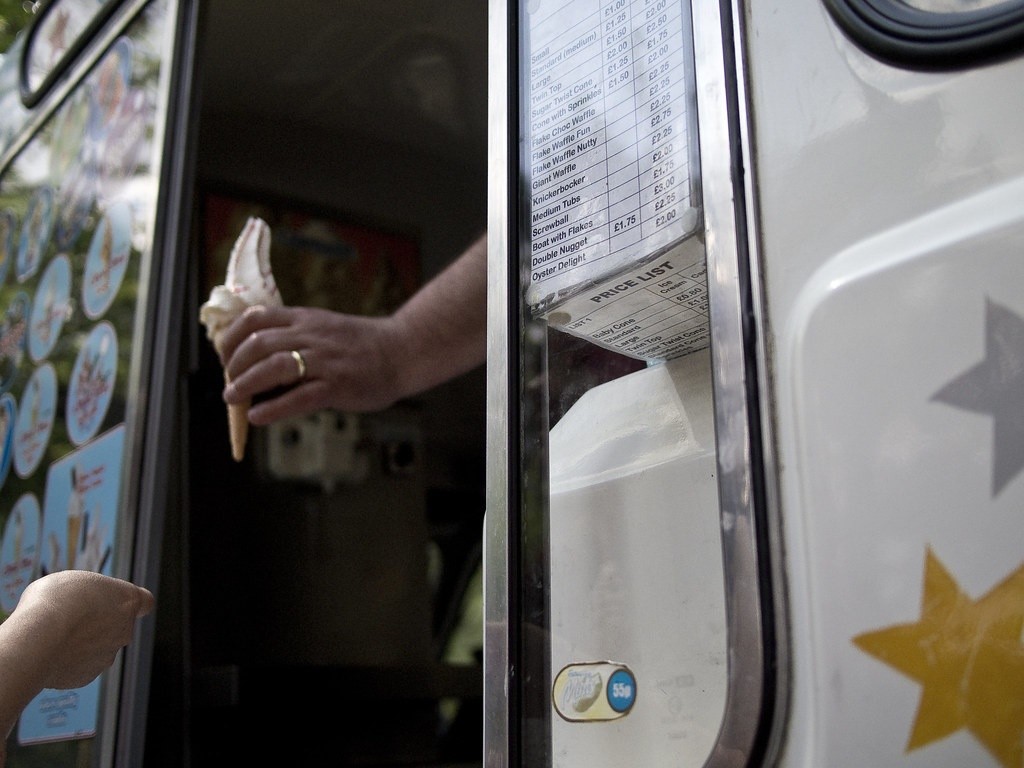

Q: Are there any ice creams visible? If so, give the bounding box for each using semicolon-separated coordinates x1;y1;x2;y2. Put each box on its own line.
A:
200;217;287;461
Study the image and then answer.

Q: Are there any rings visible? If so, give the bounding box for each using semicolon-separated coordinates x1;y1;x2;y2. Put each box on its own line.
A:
291;351;306;377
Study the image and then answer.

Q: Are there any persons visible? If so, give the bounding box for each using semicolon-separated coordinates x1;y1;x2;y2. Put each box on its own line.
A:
220;232;486;424
0;569;154;768
